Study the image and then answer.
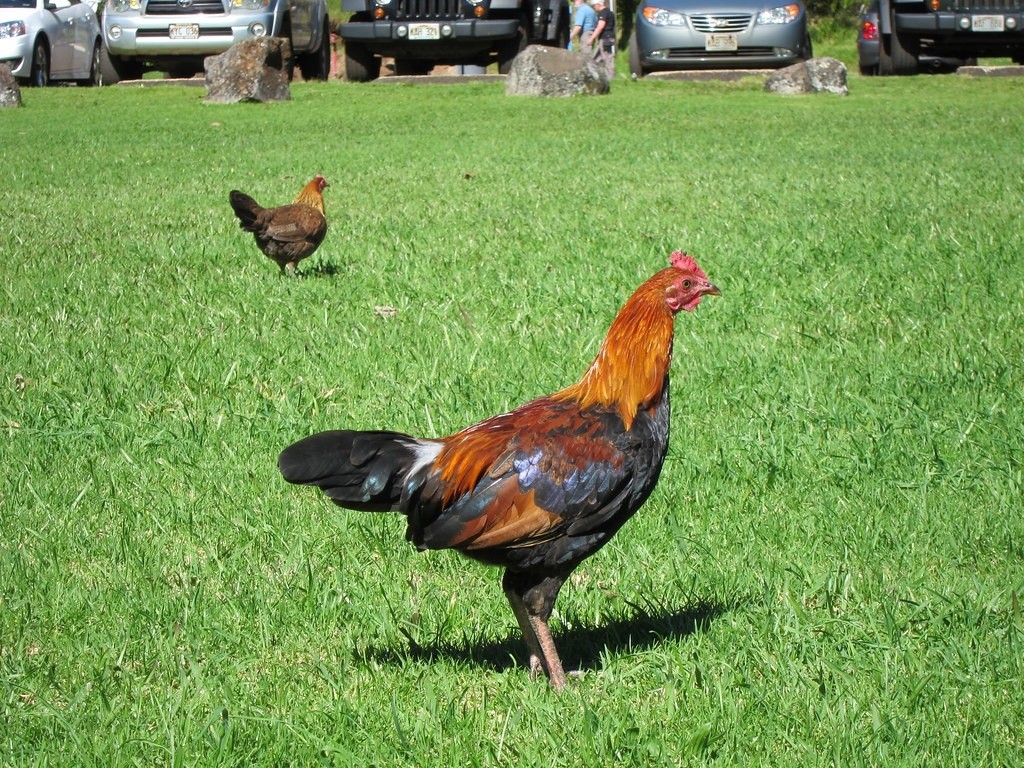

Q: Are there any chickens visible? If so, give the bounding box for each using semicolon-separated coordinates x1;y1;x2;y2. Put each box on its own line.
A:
275;250;724;692
230;173;330;280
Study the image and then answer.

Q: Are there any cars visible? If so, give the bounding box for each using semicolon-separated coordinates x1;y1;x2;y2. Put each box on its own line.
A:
859;0;978;78
100;0;331;86
0;0;104;88
628;1;813;77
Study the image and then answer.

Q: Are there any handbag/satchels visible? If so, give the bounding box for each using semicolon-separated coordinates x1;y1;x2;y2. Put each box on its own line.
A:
602;35;616;46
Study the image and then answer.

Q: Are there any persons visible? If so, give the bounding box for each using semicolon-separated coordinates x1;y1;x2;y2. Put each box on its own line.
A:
561;0;616;80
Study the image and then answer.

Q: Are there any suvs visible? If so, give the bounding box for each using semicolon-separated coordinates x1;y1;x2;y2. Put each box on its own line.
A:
876;0;1024;76
339;1;570;83
